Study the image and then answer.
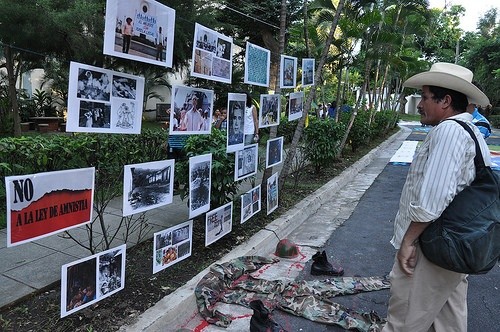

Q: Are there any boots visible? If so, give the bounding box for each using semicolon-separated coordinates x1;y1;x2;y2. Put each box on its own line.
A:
248;299;288;332
310;250;345;276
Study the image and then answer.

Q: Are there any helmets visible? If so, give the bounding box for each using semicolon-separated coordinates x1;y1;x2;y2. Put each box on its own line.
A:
276;239;296;257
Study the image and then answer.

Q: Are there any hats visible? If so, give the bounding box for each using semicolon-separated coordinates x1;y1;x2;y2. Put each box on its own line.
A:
402;62;490;109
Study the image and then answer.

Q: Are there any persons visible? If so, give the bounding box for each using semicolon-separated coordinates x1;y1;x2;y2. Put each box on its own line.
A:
467;81;492;139
285;96;289;118
76;70;138;129
69;286;96;309
230;101;242;143
270;184;276;208
317;99;350;118
174;96;211;131
121;17;164;62
380;62;492;332
212;91;260;147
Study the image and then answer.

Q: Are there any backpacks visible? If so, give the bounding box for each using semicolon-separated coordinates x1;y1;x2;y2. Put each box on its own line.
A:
419;118;500;276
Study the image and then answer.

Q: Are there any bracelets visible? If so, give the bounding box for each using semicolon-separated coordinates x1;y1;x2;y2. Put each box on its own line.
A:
255;133;259;135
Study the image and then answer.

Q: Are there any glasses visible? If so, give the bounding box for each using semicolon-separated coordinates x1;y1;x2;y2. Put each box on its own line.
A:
192;98;196;101
221;112;226;114
